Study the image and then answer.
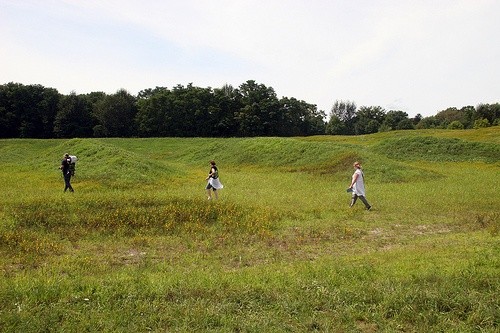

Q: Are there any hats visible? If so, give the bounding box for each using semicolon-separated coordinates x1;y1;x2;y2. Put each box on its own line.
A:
63;153;69;159
354;162;360;168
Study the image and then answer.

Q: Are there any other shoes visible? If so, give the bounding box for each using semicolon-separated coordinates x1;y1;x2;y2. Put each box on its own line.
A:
368;207;372;211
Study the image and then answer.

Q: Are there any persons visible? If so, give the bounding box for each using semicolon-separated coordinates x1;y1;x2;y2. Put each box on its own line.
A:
59;153;74;193
350;162;372;211
205;160;223;200
